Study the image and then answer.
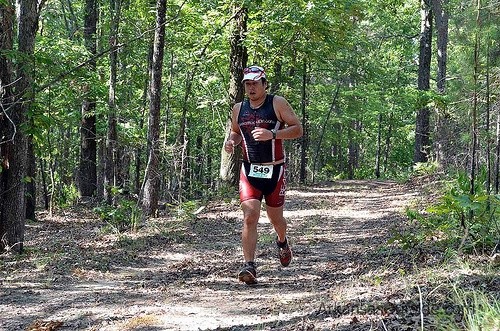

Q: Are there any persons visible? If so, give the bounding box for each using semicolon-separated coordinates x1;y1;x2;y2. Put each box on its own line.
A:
224;66;304;286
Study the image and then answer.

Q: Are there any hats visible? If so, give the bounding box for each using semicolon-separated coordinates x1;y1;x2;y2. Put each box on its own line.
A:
241;66;265;83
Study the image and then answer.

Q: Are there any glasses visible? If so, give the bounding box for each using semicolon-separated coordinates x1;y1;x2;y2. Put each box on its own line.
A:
243;67;264;73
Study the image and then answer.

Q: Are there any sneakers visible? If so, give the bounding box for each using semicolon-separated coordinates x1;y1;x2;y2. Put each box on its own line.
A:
278;239;292;266
238;267;258;285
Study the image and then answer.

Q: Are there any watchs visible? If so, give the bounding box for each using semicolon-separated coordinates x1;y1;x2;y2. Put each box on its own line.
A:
271;129;277;139
